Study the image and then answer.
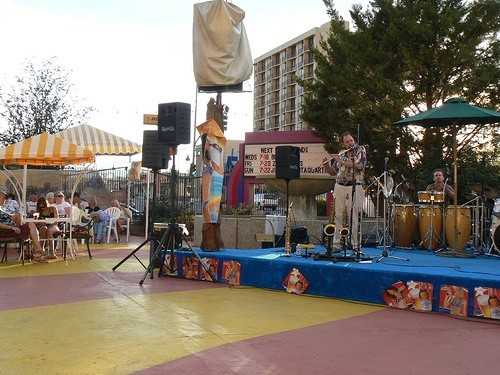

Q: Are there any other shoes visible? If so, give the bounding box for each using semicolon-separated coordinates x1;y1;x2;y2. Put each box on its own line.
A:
87;237;120;244
326;246;343;253
16;247;58;264
354;248;364;255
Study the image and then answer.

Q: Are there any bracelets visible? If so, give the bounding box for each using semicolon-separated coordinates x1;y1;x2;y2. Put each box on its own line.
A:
11;226;13;229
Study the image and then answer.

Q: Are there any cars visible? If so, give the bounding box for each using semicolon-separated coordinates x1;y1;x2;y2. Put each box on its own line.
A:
119;203;145;220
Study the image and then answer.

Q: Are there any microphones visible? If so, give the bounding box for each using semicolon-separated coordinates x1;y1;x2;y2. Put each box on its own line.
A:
384;157;389;164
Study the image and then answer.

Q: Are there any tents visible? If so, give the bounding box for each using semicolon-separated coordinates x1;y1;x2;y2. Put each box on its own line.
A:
52;123;149;242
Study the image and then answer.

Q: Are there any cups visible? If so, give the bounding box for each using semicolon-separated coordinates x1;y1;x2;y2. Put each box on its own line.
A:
477;295;490;317
287;275;299;292
410;288;419;309
32;216;37;220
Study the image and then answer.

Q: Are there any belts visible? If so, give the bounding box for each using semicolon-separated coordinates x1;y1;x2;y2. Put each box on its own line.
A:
337;180;354;186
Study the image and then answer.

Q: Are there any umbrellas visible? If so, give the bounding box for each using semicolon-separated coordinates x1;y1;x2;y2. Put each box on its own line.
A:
0;132;96;188
393;98;500;250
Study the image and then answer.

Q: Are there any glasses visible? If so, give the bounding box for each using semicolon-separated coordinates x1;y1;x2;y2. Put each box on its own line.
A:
39;205;46;208
56;195;64;197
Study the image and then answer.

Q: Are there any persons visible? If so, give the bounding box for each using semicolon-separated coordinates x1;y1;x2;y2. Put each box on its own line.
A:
0;189;72;264
322;133;366;253
425;169;455;245
83;197;105;244
65;192;81;230
109;199;131;242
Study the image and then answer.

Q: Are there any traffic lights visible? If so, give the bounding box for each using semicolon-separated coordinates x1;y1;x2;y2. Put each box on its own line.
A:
205;98;229;134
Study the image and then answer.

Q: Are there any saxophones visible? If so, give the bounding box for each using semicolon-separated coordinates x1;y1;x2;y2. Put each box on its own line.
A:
324;194;337;254
339;194;350;249
285;200;294;256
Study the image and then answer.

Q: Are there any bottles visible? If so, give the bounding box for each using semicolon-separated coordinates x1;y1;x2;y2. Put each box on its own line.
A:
488;287;500;320
296;243;302;258
290;276;304;294
418;282;432;312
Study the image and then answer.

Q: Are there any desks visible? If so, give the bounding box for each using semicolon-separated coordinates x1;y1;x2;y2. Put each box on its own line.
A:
17;218;76;262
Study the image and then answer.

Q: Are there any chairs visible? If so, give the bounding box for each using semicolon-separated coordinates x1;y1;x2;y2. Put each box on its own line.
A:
121;208;132;243
103;207;120;244
63;216;96;261
0;229;32;266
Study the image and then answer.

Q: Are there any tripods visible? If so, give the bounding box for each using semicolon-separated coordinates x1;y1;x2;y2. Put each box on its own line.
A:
417;178;489;256
112;168;169;276
140;144;217;287
364;164;409;262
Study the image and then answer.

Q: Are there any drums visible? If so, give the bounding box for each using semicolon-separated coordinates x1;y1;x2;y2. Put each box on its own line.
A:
446;205;471;249
417;191;432;203
490;197;500;254
419;205;442;250
433;191;444;203
391;204;416;248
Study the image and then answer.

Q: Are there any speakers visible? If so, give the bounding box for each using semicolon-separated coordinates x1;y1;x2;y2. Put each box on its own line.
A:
158;101;191;144
142;129;168;170
274;146;301;180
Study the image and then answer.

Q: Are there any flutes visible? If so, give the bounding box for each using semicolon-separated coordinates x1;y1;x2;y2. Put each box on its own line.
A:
318;144;357;169
